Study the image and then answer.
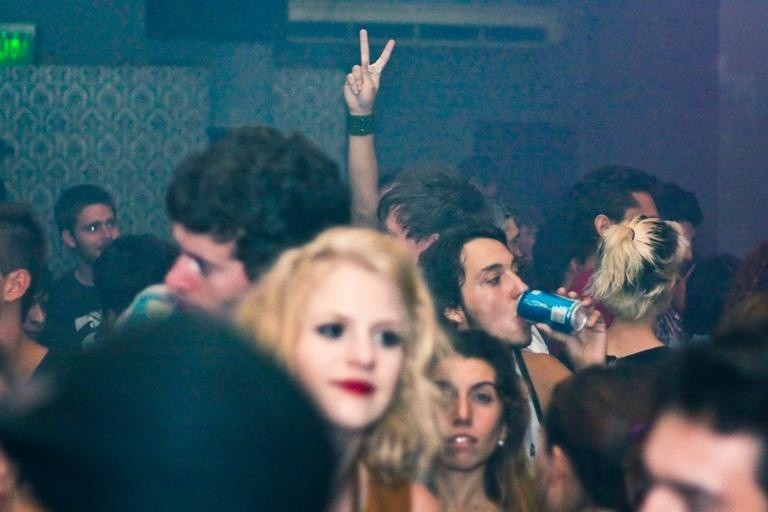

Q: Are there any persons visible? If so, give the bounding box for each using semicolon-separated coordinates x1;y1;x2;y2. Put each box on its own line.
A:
1;28;768;512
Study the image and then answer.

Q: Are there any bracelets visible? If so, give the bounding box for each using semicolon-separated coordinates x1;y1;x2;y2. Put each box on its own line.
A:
350;113;373;137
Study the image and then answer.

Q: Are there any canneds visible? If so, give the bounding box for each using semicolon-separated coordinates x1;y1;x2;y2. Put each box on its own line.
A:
514;288;588;335
